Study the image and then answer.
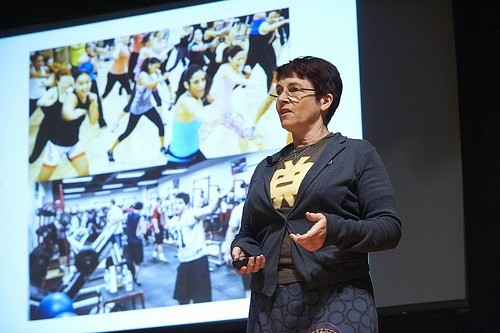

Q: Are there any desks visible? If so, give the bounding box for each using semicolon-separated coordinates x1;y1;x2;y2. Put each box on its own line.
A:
97;284;145;314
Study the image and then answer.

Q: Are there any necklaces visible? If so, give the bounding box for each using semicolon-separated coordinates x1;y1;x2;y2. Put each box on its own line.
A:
293;145;312;165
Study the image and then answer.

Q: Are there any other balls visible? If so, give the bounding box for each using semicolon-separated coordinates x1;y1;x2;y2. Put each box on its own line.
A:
54;312;78;318
40;291;73;317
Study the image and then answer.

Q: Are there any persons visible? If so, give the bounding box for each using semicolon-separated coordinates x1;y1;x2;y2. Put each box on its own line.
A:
230;56;403;333
27;6;290;321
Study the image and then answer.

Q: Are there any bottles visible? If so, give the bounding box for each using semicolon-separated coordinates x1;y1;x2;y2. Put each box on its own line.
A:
104;264;134;294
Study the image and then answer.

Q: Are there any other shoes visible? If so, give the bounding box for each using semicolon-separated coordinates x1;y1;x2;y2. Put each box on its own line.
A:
108;150;114;161
152;255;157;263
158;259;168;264
160;147;166;153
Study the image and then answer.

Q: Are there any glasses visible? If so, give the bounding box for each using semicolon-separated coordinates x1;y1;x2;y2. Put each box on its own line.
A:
269;86;316;97
